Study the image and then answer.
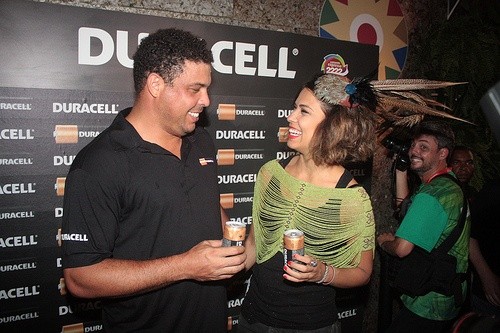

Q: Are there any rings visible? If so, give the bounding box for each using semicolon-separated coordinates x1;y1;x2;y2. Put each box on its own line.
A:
311;261;317;267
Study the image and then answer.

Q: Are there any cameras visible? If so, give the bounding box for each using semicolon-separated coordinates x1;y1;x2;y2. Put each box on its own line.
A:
382;133;412;172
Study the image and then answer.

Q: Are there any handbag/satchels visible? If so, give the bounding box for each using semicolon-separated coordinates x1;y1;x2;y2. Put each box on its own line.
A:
386;248;457;296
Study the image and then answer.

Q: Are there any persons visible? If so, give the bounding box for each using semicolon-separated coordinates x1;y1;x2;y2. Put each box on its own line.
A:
220;75;384;333
62;27;247;333
377;121;500;333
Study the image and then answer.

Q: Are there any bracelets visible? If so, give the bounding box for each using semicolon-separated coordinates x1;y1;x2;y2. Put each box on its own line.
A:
323;265;336;285
395;198;404;202
316;266;328;283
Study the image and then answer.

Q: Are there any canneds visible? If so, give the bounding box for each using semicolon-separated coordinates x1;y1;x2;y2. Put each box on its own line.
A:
221;221;246;247
283;229;304;273
